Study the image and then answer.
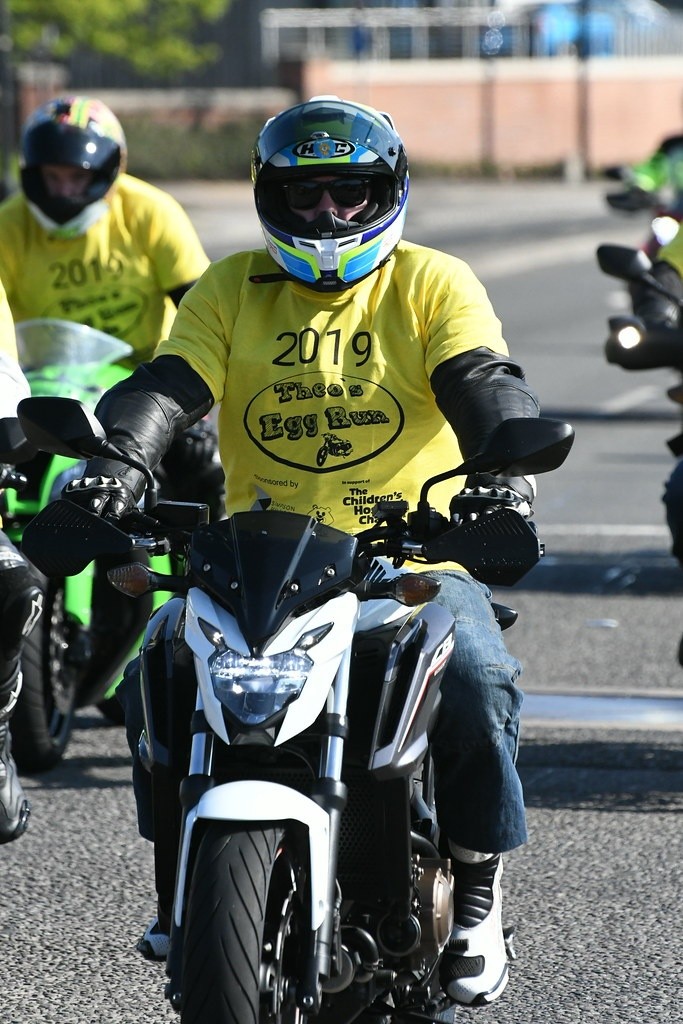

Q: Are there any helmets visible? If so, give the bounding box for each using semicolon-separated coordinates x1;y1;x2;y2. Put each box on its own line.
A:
250;95;410;292
17;96;128;241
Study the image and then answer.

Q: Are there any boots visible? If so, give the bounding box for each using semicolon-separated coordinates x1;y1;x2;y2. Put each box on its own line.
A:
0;670;30;845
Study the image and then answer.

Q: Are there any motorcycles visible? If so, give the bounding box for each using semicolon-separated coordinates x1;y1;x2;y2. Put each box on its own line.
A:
592;217;683;665
0;315;228;776
14;389;577;1024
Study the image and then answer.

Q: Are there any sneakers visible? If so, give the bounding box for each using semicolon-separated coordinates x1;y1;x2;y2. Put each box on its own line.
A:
440;839;509;1006
136;917;169;961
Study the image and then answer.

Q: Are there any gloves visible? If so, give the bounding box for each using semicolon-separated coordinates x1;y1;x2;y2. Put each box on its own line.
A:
429;344;542;523
60;354;214;529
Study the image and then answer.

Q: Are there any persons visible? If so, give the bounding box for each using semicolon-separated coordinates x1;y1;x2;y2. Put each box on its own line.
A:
0;277;45;844
626;134;683;666
0;96;227;526
58;94;541;1006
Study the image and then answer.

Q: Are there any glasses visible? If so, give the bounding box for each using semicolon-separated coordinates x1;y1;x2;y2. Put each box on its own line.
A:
281;176;373;211
